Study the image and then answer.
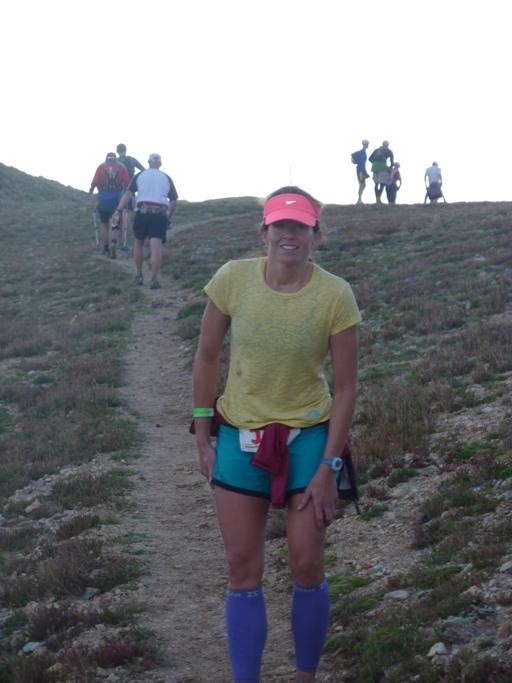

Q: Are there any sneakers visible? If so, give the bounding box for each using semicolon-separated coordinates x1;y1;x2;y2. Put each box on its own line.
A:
134;276;160;288
100;245;130;260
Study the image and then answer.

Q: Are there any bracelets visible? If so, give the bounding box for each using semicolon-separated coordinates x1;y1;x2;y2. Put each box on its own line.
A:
116;208;122;212
192;407;215;417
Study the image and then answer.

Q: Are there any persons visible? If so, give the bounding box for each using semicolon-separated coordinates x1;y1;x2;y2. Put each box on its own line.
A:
423;161;448;205
355;139;370;204
111;153;180;289
191;184;362;682
367;139;394;204
88;151;131;259
115;143;147;251
385;161;401;203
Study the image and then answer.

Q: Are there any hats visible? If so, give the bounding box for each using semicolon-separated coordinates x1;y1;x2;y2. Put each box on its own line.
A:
149;153;161;162
263;192;320;228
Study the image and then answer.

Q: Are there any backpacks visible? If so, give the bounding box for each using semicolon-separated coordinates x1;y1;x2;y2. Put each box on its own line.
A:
352;151;361;164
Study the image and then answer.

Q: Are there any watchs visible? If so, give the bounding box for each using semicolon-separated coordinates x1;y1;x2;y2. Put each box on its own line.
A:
319;455;343;471
